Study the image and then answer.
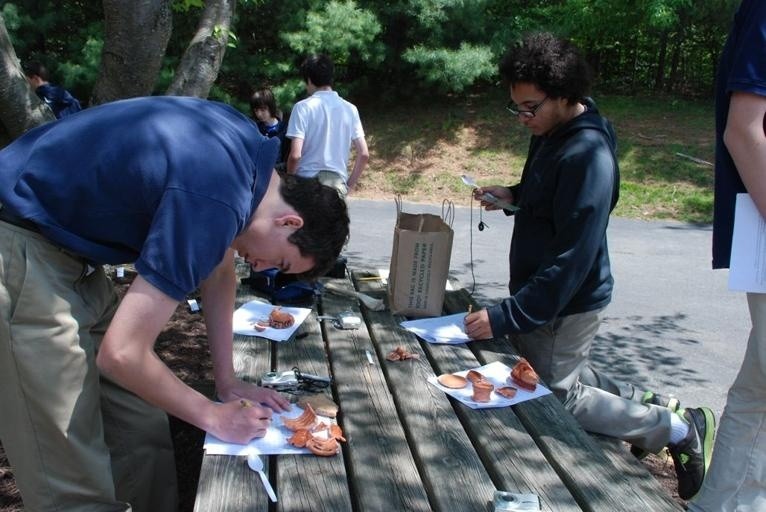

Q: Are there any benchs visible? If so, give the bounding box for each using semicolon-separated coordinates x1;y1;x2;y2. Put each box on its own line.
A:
587;433;685;511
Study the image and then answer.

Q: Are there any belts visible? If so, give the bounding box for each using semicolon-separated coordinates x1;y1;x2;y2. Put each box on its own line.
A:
0;203;99;274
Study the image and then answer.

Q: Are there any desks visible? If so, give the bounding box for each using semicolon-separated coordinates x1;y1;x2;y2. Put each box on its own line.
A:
190;265;653;511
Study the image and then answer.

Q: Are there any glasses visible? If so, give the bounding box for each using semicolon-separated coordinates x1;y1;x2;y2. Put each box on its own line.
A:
504;95;552;119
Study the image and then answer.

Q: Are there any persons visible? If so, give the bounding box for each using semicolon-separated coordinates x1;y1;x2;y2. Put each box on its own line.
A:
281;51;371;203
248;87;290;183
20;59;82;121
692;1;766;512
462;28;717;505
0;96;352;512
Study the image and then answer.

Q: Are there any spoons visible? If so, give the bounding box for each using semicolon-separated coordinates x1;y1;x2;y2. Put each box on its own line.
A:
246;451;281;505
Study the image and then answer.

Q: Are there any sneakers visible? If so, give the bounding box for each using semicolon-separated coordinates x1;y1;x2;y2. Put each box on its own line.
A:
629;390;680;459
665;405;717;500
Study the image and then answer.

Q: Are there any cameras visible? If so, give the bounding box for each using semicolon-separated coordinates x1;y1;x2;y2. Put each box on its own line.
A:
338;311;362;329
260;370;329;390
492;491;541;512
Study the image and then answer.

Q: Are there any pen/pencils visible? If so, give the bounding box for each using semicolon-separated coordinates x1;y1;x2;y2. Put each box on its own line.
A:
359;277;381;280
468;304;473;314
240;399;252;407
